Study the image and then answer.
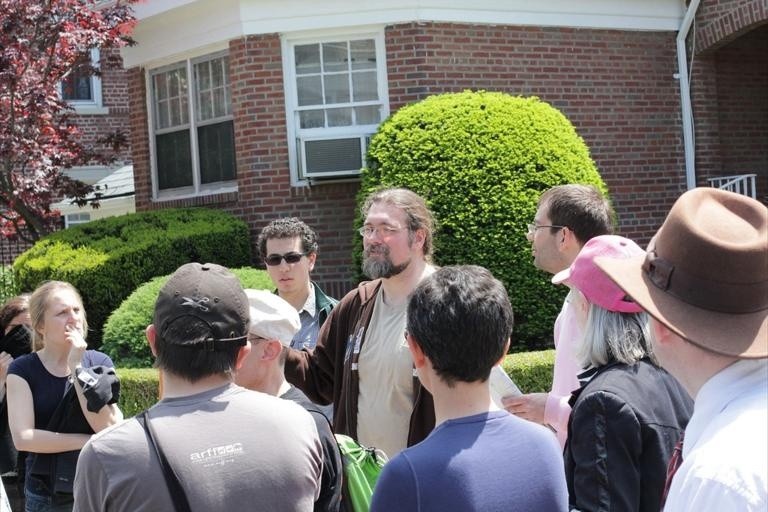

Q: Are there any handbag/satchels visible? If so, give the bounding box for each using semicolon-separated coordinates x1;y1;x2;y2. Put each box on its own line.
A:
334;428;390;512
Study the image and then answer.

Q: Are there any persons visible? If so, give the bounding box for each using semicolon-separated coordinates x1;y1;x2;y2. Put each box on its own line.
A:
0;294;34;512
551;235;694;512
370;264;569;512
284;188;442;461
500;183;616;451
6;280;124;512
73;262;324;512
234;288;345;512
257;215;341;426
592;187;768;512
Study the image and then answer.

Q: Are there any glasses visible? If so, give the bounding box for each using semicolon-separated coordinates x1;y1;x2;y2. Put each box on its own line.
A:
359;224;405;237
528;223;562;232
264;253;306;265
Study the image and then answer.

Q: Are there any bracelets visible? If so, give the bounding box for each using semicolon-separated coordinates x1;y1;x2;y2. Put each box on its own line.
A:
67;374;77;383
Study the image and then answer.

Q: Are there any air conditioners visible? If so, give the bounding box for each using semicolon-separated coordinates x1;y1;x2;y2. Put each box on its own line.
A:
301;134;367;179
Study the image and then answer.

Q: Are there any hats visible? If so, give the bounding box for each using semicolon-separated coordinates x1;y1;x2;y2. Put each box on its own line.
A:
245;289;302;347
592;189;768;358
551;234;647;313
154;262;249;350
